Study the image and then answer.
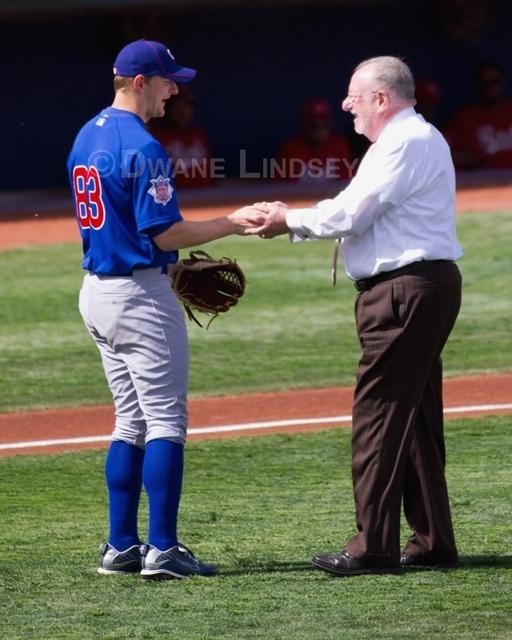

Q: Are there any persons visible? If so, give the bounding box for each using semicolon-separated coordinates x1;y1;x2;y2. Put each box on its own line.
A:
149;86;212;191
243;56;461;575
68;39;267;580
445;68;511;170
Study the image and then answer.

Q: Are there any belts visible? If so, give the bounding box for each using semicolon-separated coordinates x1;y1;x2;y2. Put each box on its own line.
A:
354;260;444;292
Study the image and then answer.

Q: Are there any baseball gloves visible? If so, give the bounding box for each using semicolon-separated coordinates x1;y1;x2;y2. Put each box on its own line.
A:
169;250;249;329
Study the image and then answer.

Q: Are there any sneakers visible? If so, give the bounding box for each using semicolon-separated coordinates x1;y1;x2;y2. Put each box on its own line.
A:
97;542;218;580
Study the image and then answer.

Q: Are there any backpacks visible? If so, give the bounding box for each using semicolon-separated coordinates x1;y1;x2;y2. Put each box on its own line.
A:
311;549;458;575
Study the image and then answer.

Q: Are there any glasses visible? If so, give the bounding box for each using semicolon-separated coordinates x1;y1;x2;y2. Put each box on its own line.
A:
348;92;376;103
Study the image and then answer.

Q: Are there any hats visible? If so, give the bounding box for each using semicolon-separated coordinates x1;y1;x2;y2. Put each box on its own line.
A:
113;39;197;84
